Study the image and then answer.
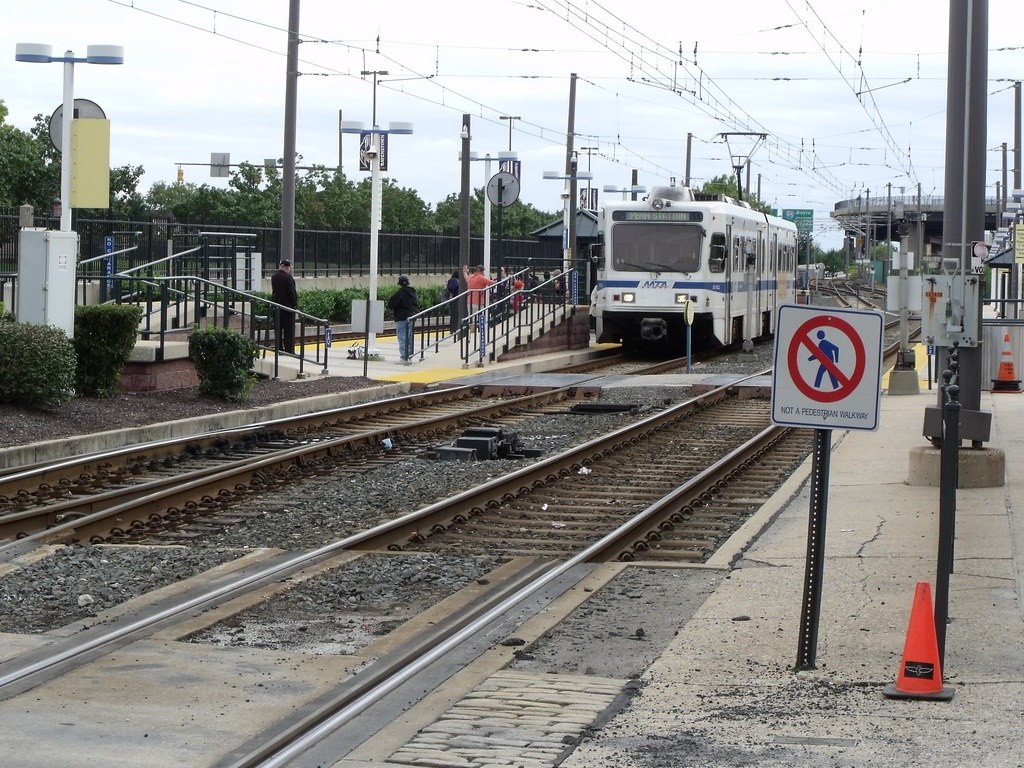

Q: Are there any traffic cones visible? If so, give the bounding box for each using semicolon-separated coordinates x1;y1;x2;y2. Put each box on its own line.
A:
881;580;955;702
988;334;1024;394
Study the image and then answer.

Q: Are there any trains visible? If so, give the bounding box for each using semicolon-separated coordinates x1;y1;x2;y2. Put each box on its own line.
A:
594;186;800;362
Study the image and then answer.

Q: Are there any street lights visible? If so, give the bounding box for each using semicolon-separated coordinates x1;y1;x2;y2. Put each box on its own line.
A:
340;119;414;361
542;170;593;304
603;184;647;201
13;41;124;233
458;151;518;345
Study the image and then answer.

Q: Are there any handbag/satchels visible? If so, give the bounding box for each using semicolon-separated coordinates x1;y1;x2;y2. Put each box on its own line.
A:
346;341;359;360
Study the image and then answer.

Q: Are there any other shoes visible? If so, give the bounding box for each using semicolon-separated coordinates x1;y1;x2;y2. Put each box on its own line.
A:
395;358;412;366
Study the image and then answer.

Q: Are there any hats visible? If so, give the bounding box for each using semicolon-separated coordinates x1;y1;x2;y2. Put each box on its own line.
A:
476;264;486;271
514;280;523;289
279;259;293;269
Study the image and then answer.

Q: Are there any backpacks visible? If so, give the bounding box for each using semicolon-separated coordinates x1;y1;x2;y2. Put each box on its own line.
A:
440;286;450;303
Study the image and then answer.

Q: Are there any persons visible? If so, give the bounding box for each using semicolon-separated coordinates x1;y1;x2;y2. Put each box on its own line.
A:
463;264;498;325
446;270;460;335
492;265;563;315
270;260;302;358
388;276;417;365
